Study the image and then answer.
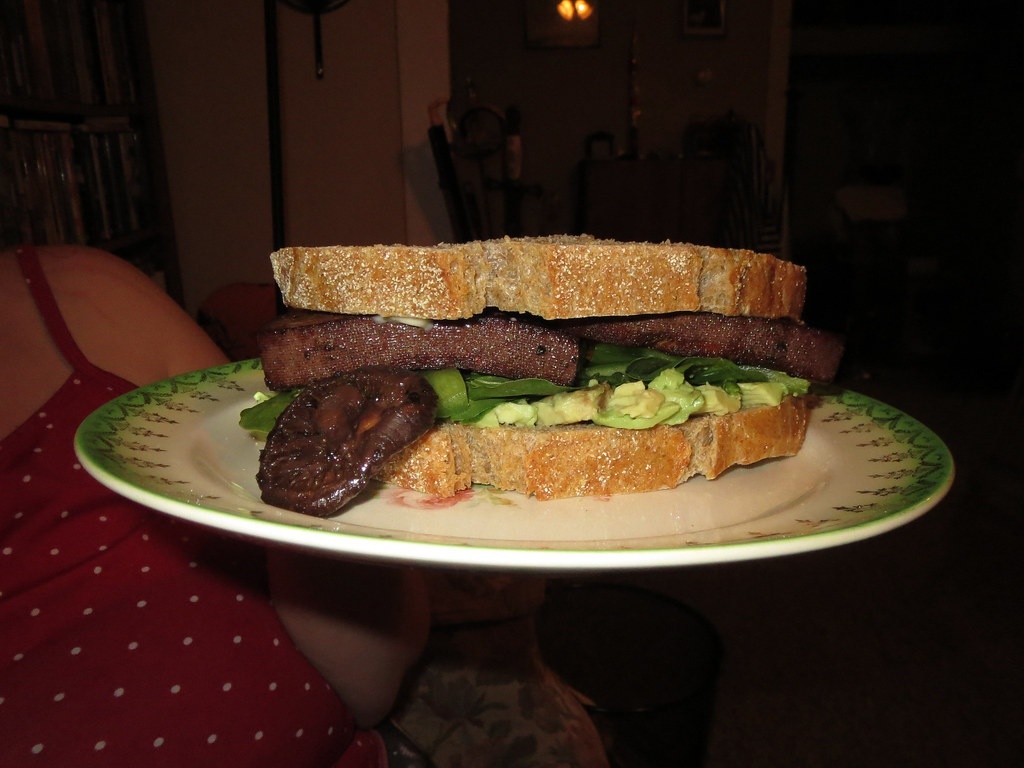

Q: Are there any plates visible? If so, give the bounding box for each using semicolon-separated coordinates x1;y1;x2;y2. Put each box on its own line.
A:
76;356;956;569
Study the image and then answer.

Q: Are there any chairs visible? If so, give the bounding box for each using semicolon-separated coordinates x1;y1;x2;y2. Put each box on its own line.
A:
425;101;786;256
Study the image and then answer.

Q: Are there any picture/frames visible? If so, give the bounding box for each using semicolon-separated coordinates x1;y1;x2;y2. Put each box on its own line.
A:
523;0;600;50
682;0;727;40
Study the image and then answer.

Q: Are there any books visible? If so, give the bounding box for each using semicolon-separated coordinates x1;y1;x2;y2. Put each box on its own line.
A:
2;0;167;293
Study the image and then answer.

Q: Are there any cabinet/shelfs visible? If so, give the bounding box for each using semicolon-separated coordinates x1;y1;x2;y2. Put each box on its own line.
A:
0;0;186;315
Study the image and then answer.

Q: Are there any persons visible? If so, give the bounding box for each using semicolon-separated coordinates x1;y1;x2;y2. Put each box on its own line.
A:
2;243;433;768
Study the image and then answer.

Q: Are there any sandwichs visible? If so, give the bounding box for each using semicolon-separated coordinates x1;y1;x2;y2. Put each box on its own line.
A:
239;230;845;498
